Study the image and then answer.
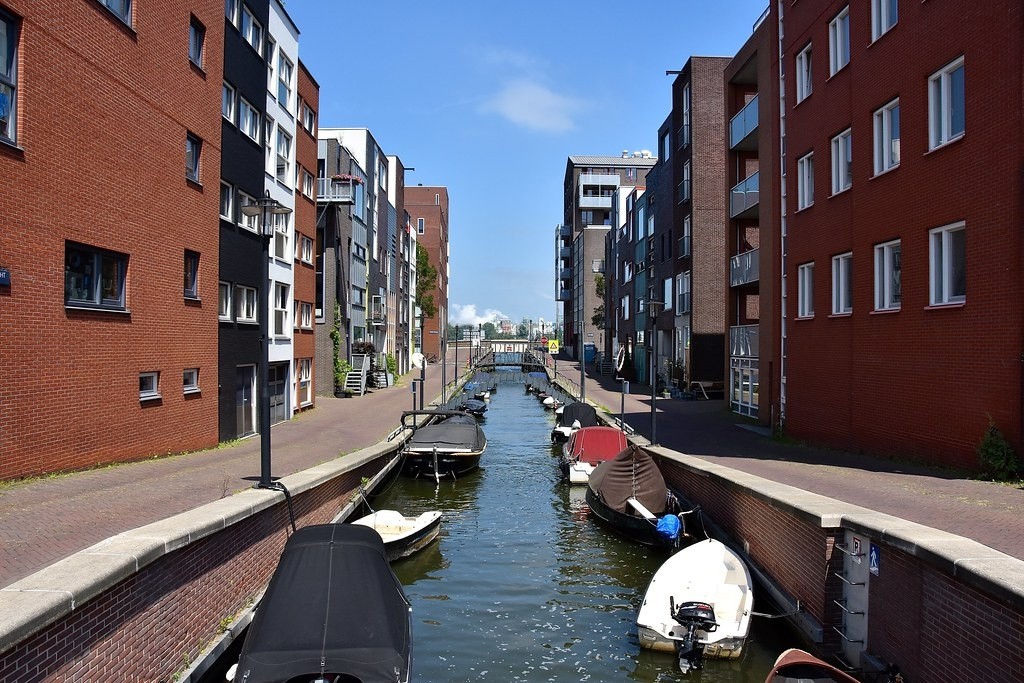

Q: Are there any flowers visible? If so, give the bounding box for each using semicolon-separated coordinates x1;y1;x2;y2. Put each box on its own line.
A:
333;174;364;185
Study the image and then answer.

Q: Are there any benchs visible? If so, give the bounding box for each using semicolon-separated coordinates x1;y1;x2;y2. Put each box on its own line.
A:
690;381;725;400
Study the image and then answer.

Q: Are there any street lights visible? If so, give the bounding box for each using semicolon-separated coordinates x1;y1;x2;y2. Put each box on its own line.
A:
641;298;669;449
239;189;294;489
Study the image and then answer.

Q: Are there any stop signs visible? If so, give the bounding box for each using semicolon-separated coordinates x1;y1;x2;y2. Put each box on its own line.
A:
541;337;547;344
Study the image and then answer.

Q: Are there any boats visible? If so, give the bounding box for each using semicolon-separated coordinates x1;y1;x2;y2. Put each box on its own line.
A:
461;373;497;413
547;401;598;444
339;509;442;565
558;423;631;486
584;442;682;547
225;523;414;683
762;648;862;683
398;410;490;481
525;370;563;410
636;536;757;677
